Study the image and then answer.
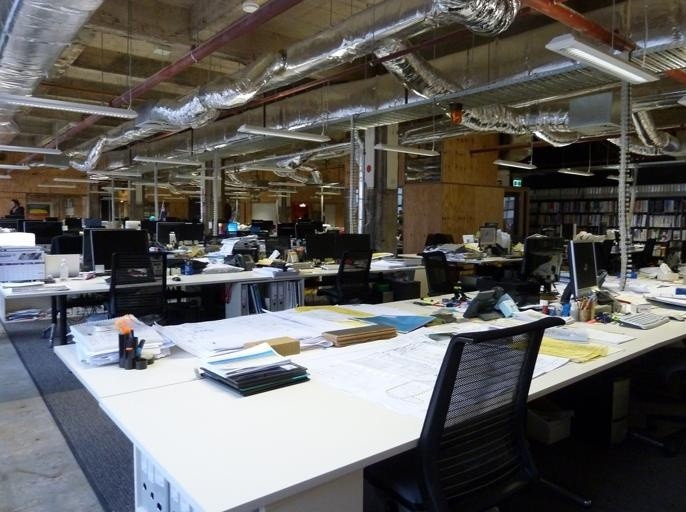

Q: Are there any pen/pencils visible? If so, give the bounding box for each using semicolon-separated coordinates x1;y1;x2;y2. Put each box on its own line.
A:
126;330;145;359
576;295;593;310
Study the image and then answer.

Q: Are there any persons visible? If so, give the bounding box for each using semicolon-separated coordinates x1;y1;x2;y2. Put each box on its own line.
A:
8;199;27;219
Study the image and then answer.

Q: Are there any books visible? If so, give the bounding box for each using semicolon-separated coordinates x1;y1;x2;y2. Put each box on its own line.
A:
197;341;309;395
321;323;397;347
528;196;686;268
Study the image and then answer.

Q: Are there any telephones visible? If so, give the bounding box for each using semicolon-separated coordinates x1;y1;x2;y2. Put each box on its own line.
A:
236;254;255;268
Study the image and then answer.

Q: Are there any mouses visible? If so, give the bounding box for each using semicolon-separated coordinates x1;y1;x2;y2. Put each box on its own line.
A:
172;277;180;281
357;264;367;268
668;314;684;321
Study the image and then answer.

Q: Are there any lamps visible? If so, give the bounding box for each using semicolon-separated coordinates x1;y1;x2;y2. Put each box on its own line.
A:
371;105;443;157
492;136;636;183
544;0;662;86
0;107;350;201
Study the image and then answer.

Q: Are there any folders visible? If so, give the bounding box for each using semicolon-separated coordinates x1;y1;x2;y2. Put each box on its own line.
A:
264;282;284;312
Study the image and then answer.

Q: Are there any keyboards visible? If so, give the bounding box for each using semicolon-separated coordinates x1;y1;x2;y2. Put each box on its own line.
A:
105;276;156;286
321;264;355;270
619;311;670;330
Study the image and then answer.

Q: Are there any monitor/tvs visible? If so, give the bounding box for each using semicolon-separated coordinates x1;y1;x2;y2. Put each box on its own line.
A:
226;222;237;231
520;234;566;295
338;233;369;260
560;239;601;302
16;216;205;277
306;233;335;258
479;226;497;245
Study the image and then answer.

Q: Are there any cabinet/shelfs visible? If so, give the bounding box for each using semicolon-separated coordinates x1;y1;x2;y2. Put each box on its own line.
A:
529;194;685;258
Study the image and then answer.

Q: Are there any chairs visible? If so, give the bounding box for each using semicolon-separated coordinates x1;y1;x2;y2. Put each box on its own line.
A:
0;214;686;512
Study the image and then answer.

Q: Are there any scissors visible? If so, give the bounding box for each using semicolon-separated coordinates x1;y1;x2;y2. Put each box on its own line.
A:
114;317;135;334
